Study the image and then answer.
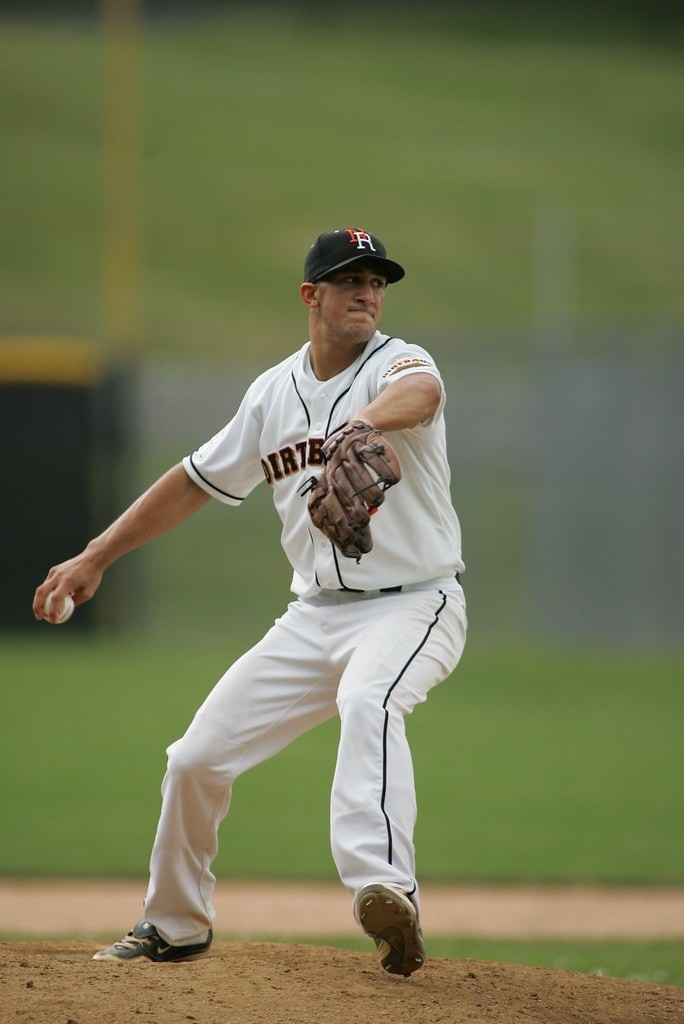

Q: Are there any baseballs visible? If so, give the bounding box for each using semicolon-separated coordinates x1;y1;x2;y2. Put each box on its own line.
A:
40;591;75;625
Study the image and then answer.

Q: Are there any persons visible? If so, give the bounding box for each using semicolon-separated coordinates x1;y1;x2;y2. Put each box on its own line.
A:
33;229;466;978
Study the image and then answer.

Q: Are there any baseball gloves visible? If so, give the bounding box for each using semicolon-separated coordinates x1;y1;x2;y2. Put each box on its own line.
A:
306;419;403;560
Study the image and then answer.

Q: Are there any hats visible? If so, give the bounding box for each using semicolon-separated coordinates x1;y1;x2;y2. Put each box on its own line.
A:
304;229;405;283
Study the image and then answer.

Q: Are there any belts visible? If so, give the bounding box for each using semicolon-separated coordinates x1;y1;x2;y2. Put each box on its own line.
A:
338;572;462;593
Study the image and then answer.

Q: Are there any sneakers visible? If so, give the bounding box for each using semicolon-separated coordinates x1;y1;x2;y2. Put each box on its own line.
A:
353;882;426;978
90;919;218;965
